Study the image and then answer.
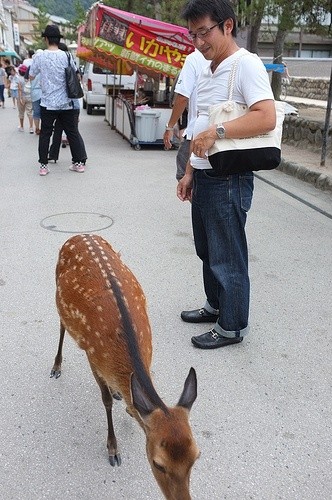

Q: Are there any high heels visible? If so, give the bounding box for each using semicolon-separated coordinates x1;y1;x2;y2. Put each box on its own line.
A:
46;153;56;164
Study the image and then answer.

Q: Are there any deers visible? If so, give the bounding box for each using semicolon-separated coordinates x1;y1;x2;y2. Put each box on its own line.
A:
50;234;201;500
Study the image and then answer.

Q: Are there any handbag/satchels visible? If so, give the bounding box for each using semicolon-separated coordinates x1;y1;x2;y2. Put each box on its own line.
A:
65;50;84;98
208;54;302;175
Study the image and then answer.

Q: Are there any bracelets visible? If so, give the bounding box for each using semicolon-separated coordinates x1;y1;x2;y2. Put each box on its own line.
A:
166;128;173;130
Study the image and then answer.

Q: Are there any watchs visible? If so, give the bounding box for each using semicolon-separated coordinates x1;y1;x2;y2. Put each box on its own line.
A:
216;123;225;139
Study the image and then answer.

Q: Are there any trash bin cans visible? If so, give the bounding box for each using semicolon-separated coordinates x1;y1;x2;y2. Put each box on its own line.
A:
134;109;161;142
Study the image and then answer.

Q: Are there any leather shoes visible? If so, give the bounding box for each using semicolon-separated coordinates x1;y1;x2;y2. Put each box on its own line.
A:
191;325;242;348
181;307;222;323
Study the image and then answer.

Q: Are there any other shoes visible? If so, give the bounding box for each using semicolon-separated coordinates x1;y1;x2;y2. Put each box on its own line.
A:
29;128;33;133
35;129;41;135
17;125;24;132
39;164;48;175
70;161;85;172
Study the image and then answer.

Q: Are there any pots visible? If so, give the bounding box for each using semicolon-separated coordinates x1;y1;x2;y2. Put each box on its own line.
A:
154;90;167;102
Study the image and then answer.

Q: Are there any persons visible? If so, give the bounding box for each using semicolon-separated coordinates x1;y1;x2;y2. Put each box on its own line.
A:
176;0;277;349
0;49;43;134
30;25;87;176
48;43;80;163
163;47;212;181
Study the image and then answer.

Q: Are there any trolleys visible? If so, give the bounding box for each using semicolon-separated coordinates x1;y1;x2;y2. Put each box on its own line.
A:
76;3;187;150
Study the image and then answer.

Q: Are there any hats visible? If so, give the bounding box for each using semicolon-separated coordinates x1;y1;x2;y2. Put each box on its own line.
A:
18;64;28;73
41;25;64;39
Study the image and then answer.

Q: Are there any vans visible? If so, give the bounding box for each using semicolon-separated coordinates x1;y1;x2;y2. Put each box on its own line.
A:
78;58;136;115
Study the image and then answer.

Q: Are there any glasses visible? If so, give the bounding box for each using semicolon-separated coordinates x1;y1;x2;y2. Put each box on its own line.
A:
189;18;228;41
